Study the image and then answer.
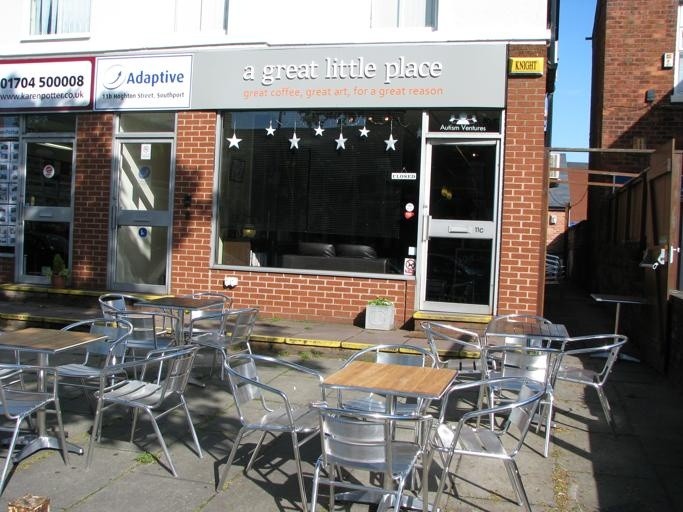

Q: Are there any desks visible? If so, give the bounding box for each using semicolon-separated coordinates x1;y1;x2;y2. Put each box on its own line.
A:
589;291;654;363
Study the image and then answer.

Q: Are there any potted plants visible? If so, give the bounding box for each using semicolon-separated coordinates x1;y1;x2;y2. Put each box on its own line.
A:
47;255;69;289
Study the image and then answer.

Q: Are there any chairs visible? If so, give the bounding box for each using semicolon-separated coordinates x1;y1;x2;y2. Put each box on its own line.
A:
189;307;260;383
46;317;134;442
483;313;555;351
103;309;182;380
419;319;496;432
98;293;181;379
308;401;434;510
535;332;629;443
215;353;327;512
0;344;70;497
84;343;204;478
482;346;564;461
162;292;232;377
419;375;547;512
